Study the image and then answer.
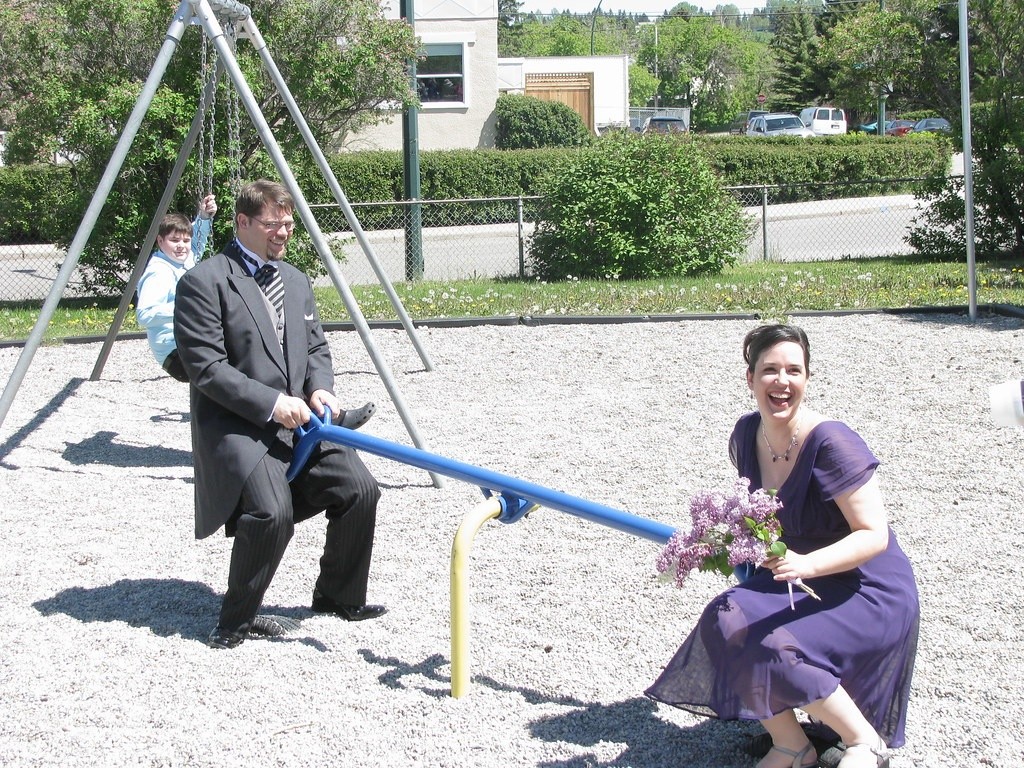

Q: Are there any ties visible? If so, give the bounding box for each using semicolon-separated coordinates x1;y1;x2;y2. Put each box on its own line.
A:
235;243;286;318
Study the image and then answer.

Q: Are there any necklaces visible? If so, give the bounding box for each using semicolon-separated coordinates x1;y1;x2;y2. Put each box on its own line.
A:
762;406;802;462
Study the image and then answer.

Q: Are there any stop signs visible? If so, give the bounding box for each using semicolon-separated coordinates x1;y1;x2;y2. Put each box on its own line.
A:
757;95;765;103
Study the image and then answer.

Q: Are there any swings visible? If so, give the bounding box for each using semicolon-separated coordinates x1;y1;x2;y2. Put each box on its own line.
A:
196;0;244;268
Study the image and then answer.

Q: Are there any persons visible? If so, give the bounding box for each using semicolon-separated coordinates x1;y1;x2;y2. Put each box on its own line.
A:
643;324;920;768
175;179;387;649
138;195;378;430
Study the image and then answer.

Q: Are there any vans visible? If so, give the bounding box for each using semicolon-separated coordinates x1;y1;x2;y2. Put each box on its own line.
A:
747;110;770;126
799;106;847;137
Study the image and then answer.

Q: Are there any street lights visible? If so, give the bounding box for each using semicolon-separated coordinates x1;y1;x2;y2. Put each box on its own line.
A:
635;22;658;116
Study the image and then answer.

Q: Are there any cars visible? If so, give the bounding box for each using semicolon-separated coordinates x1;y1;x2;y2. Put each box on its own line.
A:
913;119;951;138
729;114;747;135
854;119;918;136
745;115;816;139
635;117;694;135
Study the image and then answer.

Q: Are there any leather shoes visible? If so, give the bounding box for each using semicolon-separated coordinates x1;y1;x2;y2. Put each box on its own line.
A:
208;622;247;650
310;588;388;621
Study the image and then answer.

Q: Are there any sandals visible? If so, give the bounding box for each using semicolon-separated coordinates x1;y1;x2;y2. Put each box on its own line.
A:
340;402;377;431
773;736;819;768
846;738;887;768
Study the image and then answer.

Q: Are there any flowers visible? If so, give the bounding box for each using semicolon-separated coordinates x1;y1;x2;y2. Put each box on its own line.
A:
654;476;821;611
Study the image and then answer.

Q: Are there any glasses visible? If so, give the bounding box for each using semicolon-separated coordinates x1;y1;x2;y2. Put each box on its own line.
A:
249;215;295;231
